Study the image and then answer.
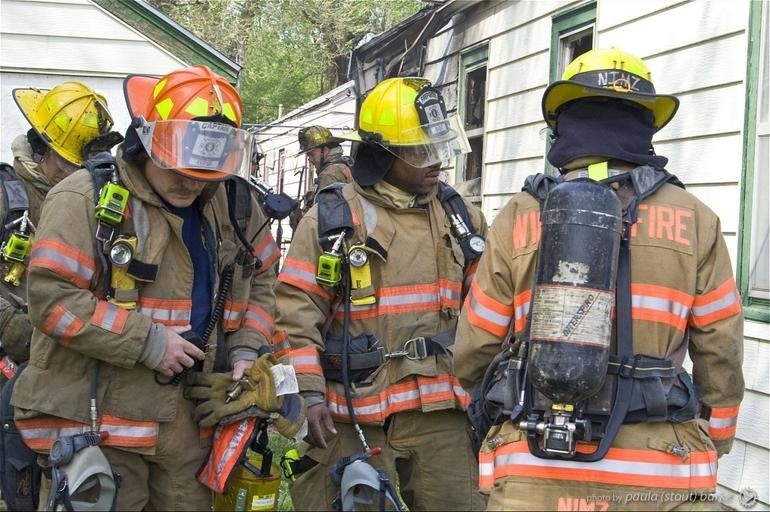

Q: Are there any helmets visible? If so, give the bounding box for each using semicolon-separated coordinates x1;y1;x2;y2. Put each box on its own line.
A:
295;76;472;158
123;64;244;182
12;81;114;169
541;49;680;133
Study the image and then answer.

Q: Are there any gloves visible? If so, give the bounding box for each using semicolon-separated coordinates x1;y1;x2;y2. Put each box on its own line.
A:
0;306;35;363
184;352;307;438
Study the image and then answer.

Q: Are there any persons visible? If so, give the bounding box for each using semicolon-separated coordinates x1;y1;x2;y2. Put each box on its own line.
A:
0;48;745;512
